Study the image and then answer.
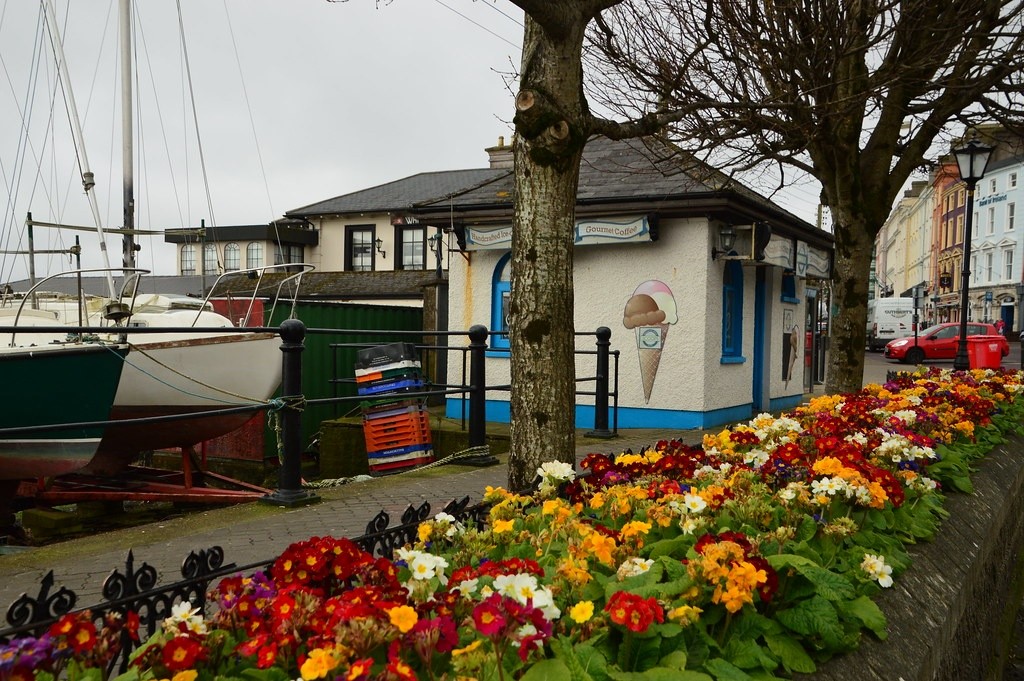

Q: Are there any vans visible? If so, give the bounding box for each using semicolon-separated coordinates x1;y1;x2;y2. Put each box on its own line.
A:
866;297;922;352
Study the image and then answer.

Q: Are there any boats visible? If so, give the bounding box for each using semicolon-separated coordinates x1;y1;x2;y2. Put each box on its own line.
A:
0;0;316;479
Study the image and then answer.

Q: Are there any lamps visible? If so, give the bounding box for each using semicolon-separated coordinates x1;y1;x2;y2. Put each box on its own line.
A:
712;220;737;260
428;236;436;251
374;237;385;258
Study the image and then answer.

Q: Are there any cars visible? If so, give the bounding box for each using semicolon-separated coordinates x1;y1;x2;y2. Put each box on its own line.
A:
882;320;1011;365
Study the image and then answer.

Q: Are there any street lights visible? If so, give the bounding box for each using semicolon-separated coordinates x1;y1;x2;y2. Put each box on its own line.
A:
946;131;999;371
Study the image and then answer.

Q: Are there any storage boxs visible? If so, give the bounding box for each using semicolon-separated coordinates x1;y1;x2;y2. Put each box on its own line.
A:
354;341;436;477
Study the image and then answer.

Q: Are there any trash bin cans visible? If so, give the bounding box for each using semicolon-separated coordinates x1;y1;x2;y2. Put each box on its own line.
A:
955;334;978;367
975;336;1001;369
